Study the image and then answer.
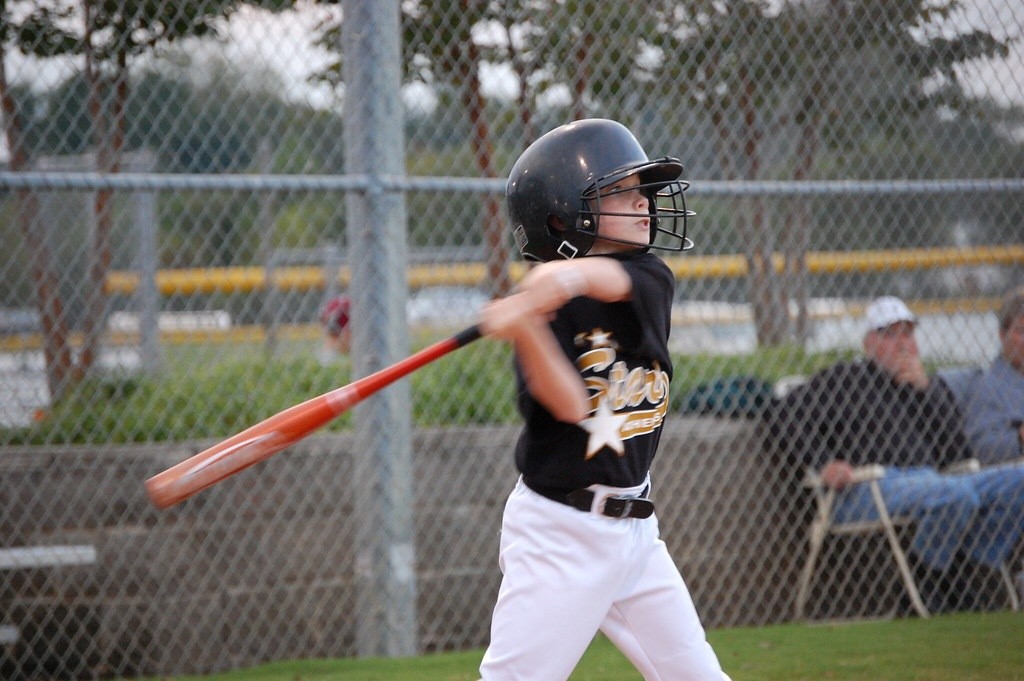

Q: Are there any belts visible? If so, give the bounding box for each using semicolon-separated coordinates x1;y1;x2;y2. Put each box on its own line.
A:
522;474;654;519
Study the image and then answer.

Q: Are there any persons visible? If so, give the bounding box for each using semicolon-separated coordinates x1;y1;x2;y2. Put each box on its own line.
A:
481;117;730;681
760;294;1024;619
959;288;1024;471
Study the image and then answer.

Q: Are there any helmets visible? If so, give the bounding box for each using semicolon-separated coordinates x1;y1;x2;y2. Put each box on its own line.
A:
505;117;694;263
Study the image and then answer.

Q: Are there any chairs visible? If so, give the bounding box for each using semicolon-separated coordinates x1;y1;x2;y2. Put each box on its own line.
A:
773;374;1021;622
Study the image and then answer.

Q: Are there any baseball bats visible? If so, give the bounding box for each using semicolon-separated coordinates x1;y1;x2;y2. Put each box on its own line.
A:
145;320;488;510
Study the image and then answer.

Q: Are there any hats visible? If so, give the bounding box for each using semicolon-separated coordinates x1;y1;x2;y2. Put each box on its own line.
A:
860;295;917;338
